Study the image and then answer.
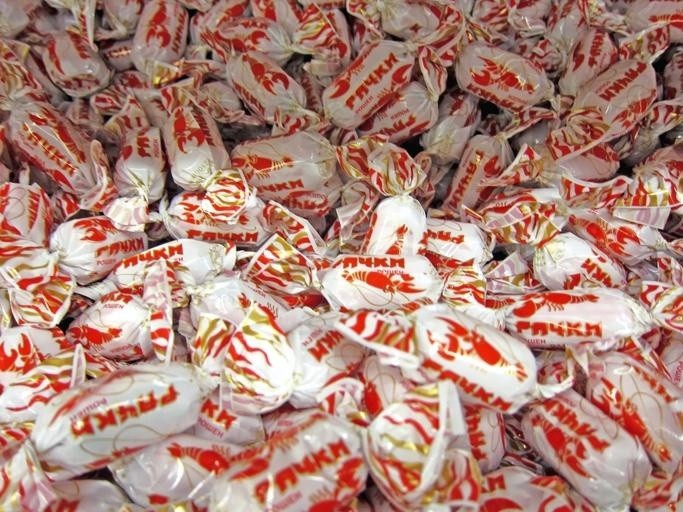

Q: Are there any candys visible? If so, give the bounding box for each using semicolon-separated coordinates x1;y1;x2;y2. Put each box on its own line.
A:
0;0;683;511
